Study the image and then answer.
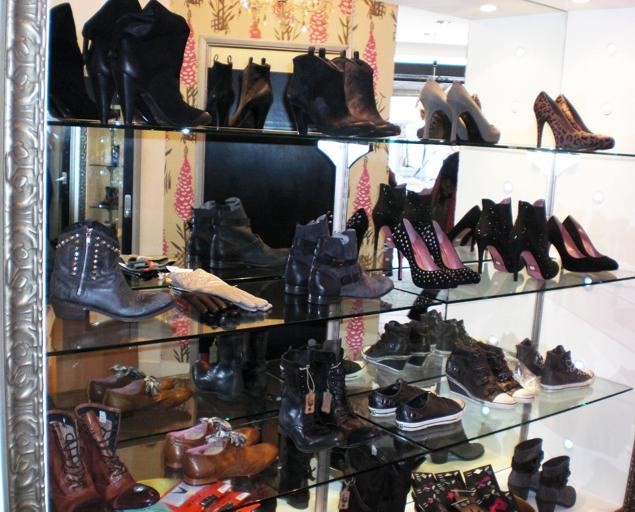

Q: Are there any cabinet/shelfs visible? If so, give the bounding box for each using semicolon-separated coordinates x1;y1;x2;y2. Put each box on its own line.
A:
84;128;123;251
44;110;635;512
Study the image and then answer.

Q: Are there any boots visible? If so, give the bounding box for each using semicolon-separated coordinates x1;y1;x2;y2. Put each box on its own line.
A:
278;339;380;454
508;438;576;512
193;330;269;403
46;402;160;512
516;337;595;390
420;309;478;355
277;435;315;509
337;451;426;512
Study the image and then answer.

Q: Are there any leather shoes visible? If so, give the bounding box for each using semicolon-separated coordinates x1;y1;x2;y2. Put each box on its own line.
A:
307;228;394;305
165;416;279;485
187;198;290;269
89;364;193;419
284;214;330;295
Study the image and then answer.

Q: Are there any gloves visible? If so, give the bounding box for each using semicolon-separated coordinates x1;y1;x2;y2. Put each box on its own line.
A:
177;267;273;318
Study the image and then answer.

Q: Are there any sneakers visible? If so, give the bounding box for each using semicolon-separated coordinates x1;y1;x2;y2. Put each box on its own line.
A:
368;377;466;432
342;358;362;381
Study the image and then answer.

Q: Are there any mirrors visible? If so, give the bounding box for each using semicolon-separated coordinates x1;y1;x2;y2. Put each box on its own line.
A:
189;34;353;376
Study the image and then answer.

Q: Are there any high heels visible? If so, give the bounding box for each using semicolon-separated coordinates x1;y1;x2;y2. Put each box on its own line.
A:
287;46;401;138
448;196;618;281
209;54;273;129
407;421;484;463
49;225;175;321
361;320;431;371
417;78;500;143
49;0;211;128
533;91;614;149
446;342;535;409
347;209;368;259
371;184;480;289
